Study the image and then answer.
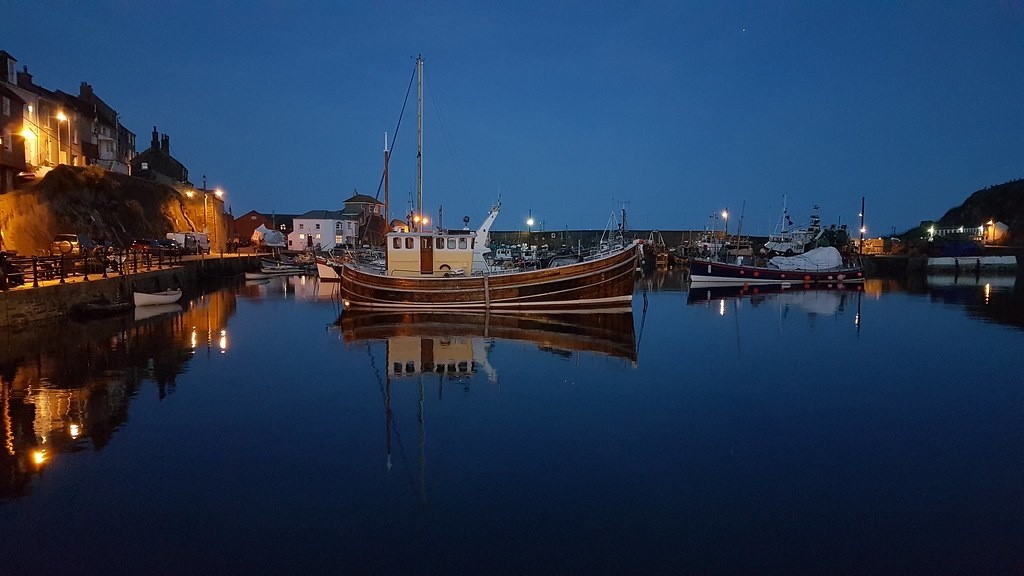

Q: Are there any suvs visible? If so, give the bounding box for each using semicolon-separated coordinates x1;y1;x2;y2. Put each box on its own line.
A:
51;234;81;257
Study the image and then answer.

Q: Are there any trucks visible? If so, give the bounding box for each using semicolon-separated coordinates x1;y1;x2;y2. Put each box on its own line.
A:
166;232;199;255
180;232;212;256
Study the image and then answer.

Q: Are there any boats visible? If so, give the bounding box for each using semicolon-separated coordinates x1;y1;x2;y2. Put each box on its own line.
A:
244;272;272;281
644;192;868;291
245;279;270;286
259;241;385;283
133;288;183;306
133;303;183;321
493;208;624;268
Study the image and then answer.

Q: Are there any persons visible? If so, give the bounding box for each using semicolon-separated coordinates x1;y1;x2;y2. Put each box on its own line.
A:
226;240;238;255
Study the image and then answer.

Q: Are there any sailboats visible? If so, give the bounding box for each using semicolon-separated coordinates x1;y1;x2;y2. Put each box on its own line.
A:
334;53;640;313
324;303;633;503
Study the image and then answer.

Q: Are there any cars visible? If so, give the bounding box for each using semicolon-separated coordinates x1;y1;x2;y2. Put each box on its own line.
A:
129;238;184;256
